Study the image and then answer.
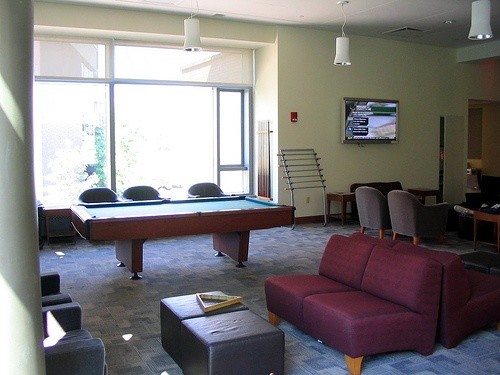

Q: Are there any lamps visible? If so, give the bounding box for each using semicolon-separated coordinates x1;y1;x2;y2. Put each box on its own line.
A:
334;1;352;66
184;0;202;51
468;0;494;40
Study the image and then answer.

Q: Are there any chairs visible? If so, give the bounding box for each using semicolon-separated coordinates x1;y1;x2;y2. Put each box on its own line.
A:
355;186;390;239
187;183;230;198
78;186;120;204
120;186;166;201
387;190;449;245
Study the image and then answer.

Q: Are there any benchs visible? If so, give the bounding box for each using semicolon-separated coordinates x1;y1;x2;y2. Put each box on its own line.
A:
264;235;500;375
352;182;424;224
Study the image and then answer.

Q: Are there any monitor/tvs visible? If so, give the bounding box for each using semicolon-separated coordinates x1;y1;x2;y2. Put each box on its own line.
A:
340;97;400;144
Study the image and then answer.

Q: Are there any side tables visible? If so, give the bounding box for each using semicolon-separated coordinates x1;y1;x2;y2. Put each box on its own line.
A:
409;189;439;205
44;208;78;245
328;191;358;225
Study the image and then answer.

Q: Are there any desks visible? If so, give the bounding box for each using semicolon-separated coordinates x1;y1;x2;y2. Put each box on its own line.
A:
70;194;297;280
472;210;500;253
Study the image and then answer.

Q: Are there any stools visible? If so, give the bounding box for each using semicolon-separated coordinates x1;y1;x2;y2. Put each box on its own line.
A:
459;251;500;274
159;290;285;375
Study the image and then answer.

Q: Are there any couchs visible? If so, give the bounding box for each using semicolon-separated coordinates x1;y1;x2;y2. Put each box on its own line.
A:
41;273;109;375
